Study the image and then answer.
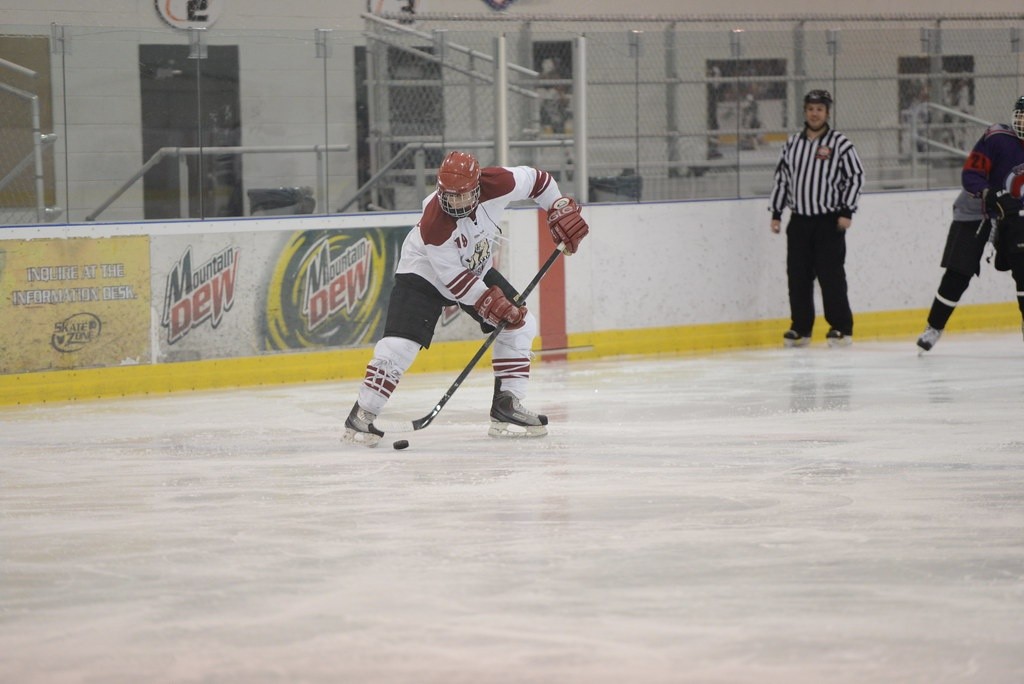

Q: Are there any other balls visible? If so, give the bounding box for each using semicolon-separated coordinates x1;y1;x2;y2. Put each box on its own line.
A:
392;440;409;450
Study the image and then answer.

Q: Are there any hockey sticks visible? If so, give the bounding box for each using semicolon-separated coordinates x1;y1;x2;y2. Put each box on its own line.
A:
372;241;566;433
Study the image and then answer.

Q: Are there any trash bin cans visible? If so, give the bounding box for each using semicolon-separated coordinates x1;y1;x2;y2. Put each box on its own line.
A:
248;186;315;215
589;175;643;202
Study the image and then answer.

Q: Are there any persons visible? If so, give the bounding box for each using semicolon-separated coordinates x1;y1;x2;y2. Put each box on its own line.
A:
342;152;589;449
533;55;971;169
917;96;1024;355
767;88;864;346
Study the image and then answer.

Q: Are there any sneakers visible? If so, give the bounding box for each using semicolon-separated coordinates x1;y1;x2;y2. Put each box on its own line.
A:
487;378;550;437
784;330;811;348
340;401;384;448
916;325;942;358
826;327;853;351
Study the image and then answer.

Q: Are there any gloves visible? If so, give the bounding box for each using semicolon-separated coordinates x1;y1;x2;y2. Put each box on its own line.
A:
474;286;528;330
547;197;590;257
984;188;1013;220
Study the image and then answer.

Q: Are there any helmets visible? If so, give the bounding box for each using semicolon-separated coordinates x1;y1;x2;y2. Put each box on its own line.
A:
435;151;481;218
805;90;832;105
1011;96;1024;139
